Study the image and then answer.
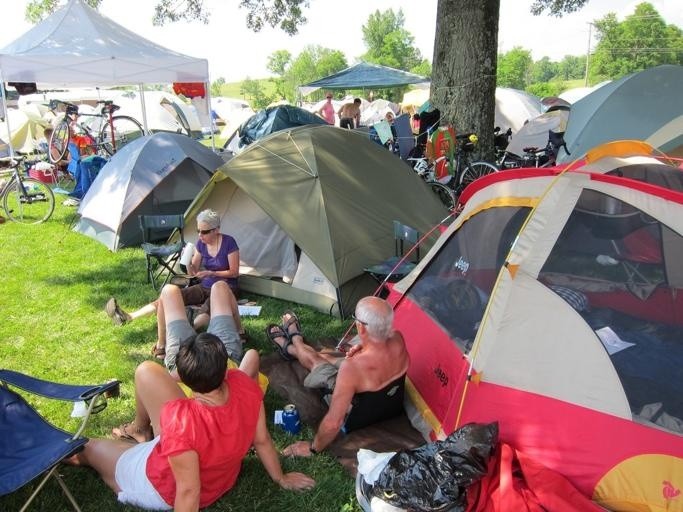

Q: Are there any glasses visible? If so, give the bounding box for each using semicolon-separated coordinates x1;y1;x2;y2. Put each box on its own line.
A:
327;97;331;98
197;228;216;234
351;311;368;326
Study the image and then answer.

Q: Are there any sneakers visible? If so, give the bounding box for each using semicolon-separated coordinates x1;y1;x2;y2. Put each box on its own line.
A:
106;297;128;326
186;306;194;329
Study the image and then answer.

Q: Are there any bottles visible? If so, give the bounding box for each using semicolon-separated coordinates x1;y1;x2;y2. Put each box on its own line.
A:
283;405;300;435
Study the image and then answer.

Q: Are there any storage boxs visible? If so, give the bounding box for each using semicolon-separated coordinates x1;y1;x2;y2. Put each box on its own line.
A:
28;164;61;184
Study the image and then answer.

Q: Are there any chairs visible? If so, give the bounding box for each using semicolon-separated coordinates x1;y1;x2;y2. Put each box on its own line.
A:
137;214;186;291
364;221;420;299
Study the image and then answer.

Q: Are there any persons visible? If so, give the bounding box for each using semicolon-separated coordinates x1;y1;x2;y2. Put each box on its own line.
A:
319;92;335;126
106;209;240;327
55;330;316;512
265;295;410;457
151;280;269;398
337;98;361;129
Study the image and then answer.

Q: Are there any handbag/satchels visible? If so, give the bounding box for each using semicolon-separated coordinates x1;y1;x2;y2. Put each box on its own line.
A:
170;273;201;289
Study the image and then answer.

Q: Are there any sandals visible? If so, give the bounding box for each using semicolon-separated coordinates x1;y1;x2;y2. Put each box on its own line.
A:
266;324;293;360
281;309;304;346
240;325;248;344
150;344;165;361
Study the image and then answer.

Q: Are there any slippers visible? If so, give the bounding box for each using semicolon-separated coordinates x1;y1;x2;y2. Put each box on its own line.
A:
112;422;138;443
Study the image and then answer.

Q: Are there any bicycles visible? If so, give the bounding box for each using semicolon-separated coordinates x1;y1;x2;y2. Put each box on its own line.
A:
406;156;456;214
497;138;556;170
46;101;145;164
1;155;56;227
454;132;500;193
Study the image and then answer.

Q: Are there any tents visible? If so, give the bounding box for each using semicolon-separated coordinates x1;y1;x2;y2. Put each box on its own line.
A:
494;86;543;137
212;96;256;141
556;62;683;168
0;99;52;156
302;99;341;128
364;98;400;126
152;124;455;320
223;103;329;158
503;106;570;170
297;60;431;126
0;0;215;223
337;94;370;126
558;79;612;105
18;90;202;137
335;139;683;512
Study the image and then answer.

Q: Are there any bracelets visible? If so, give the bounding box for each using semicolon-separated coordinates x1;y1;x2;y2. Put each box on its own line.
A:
309;441;316;454
